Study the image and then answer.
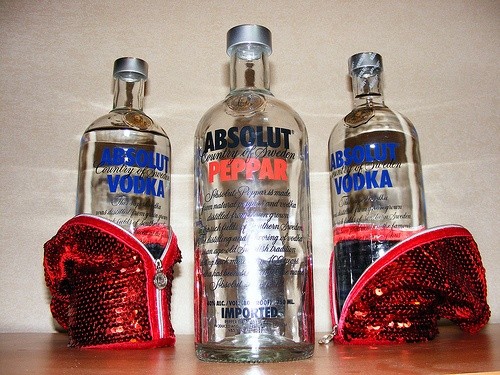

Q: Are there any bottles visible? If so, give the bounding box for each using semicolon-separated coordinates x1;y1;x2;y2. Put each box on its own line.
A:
328;52;427;321
193;24;314;364
75;56;171;262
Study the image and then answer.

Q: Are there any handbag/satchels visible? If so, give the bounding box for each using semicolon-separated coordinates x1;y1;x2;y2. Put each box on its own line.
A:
319;225;490;347
43;213;180;351
